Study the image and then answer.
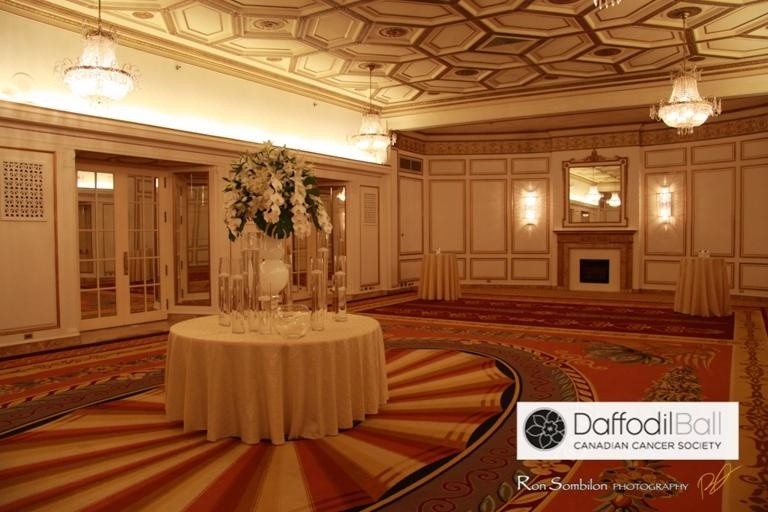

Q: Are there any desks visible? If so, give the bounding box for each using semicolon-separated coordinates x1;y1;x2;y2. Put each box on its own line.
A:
418;252;462;301
673;256;732;318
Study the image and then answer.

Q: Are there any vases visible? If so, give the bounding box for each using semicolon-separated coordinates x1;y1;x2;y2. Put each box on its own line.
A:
258;232;289;334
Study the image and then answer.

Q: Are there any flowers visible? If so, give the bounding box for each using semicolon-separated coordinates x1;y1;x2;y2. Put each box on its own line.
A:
221;139;334;243
165;310;389;445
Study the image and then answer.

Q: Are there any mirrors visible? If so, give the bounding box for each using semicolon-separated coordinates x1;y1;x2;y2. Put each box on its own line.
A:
562;157;628;227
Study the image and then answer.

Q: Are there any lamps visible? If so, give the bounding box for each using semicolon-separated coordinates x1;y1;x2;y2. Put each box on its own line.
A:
605;176;622;209
346;63;397;153
519;181;542;236
585;166;604;205
649;6;722;136
52;0;143;105
656;175;672;232
336;186;345;202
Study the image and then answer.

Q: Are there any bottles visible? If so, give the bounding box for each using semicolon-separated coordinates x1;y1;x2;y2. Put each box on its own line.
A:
270;304;312;339
